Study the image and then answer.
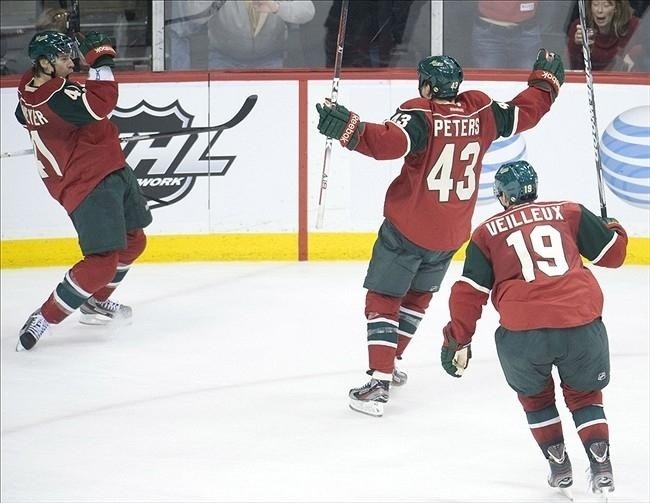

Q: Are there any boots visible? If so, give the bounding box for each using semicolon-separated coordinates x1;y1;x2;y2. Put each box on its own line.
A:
349;317;399;403
391;304;427;383
80;263;132;320
19;270;94;351
572;406;615;493
526;404;573;488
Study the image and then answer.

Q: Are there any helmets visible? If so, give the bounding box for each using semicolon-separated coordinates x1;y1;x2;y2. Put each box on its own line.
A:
494;161;539;210
28;31;77;67
417;56;463;97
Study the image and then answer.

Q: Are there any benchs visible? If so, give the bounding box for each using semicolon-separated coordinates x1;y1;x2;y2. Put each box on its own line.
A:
0;0;167;75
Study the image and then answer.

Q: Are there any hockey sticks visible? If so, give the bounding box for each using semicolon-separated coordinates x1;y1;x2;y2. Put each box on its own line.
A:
1;95;256;158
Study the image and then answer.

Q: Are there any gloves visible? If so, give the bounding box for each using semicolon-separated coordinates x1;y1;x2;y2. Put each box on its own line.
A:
528;47;565;103
316;99;366;151
441;322;471;378
80;31;118;68
598;216;628;242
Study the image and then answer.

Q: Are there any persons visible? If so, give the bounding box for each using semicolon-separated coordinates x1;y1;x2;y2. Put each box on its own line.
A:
316;45;566;417
14;29;154;354
441;158;628;503
0;0;649;80
